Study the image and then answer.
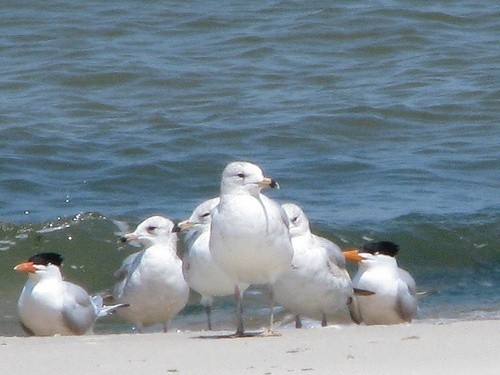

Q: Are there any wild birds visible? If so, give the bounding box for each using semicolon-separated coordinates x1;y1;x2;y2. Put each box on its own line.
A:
171;196;254;330
273;203;355;329
12;252;131;336
209;161;295;338
342;240;420;327
113;215;190;334
